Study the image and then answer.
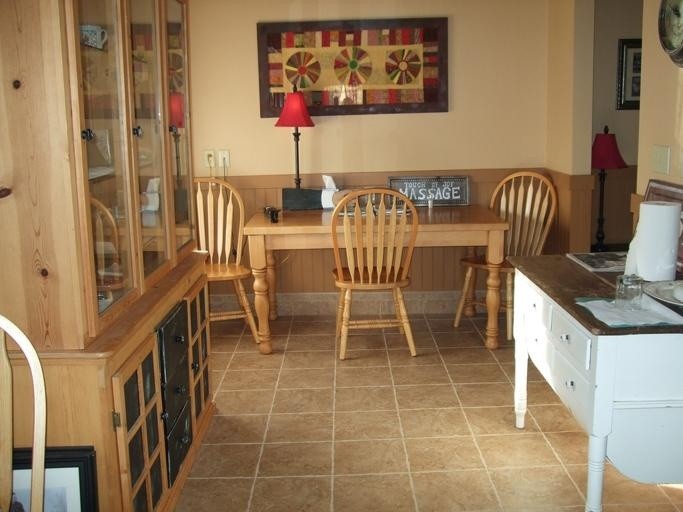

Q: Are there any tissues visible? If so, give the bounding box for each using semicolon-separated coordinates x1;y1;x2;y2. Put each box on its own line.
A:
142;177;161;211
320;174;340;210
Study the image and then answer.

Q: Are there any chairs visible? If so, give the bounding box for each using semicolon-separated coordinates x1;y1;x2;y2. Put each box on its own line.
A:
452;171;559;341
195;177;261;354
329;188;422;359
88;193;130;305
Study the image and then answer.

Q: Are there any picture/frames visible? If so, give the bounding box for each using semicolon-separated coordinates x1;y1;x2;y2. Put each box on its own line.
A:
256;16;449;119
616;37;643;110
636;178;683;273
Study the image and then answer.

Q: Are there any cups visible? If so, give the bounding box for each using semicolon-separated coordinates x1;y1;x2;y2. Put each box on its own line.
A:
616;275;643;312
80;25;108;49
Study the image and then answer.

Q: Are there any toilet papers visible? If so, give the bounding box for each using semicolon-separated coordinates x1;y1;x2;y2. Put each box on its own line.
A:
623;201;682;281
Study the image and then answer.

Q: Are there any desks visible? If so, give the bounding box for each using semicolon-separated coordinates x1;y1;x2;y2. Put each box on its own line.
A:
242;204;511;356
504;252;683;512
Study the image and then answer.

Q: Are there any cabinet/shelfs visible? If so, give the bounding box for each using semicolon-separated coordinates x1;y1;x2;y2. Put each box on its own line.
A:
157;0;198;265
0;0;173;350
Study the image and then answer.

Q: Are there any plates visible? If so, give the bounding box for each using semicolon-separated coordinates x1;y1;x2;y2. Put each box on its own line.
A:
643;280;683;307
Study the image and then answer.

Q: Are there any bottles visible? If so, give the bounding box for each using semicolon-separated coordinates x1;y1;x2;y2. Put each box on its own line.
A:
428;197;433;208
270;209;278;223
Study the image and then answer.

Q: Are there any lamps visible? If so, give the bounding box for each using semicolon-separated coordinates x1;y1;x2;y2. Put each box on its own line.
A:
166;91;187;212
275;92;320;211
593;124;630;252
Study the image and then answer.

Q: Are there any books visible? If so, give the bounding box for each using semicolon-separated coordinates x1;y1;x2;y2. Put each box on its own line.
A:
339;205;411;216
